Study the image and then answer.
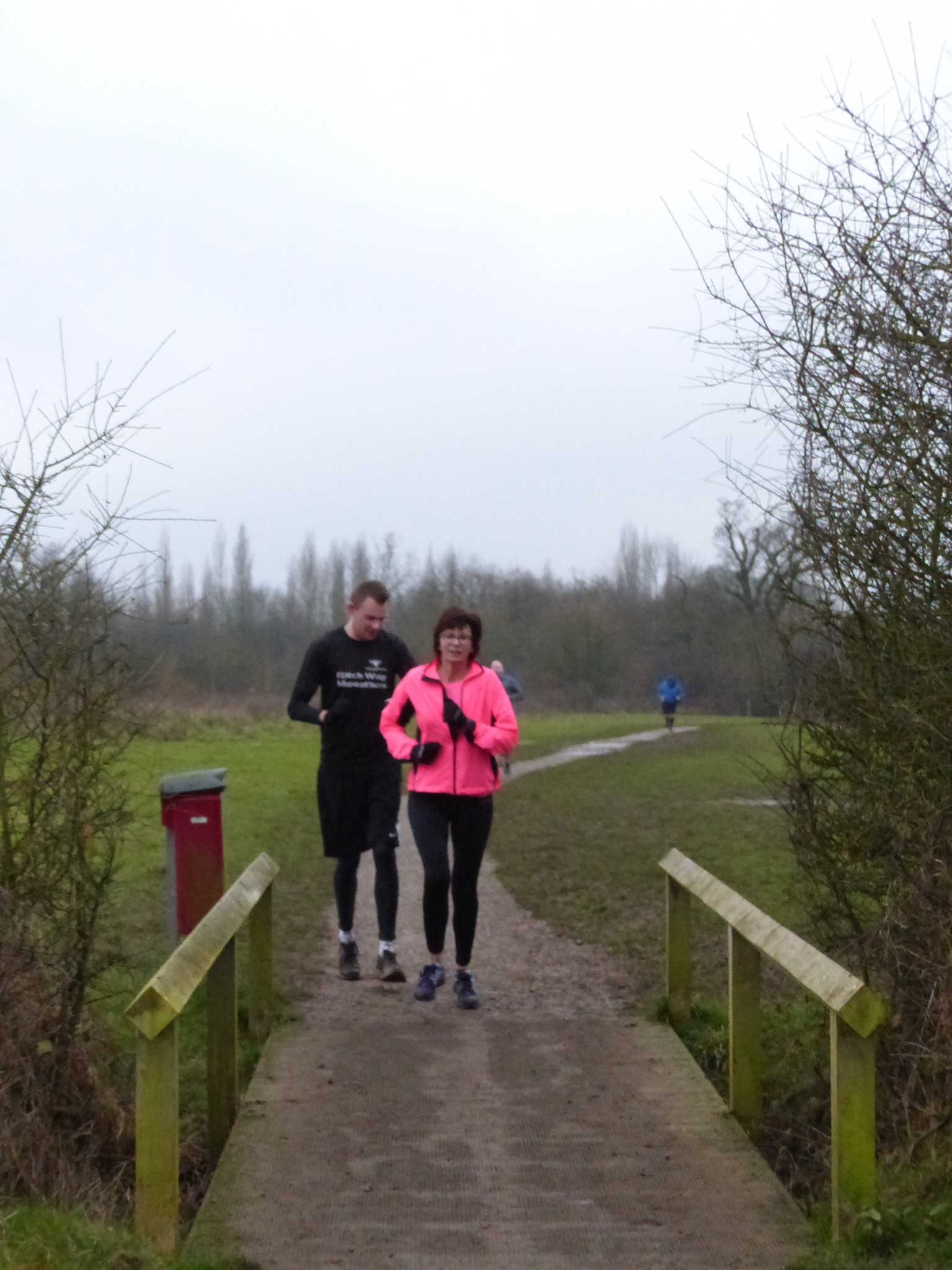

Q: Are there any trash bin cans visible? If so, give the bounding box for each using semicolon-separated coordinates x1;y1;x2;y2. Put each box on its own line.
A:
152;764;228;939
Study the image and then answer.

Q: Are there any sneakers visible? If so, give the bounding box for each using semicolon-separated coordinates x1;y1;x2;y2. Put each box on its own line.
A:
338;941;360;979
376;950;406;982
453;971;480;1006
413;964;445;1001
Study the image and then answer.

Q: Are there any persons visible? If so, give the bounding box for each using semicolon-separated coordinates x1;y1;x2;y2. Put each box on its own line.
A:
377;606;518;1010
287;580;426;984
658;673;685;730
487;659;523;773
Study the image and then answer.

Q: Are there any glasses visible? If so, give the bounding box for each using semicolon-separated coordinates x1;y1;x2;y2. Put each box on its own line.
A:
438;631;473;643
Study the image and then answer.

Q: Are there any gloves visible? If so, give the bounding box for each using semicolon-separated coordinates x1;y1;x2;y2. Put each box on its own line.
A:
442;697;476;742
324;696;352;723
410;742;441;763
